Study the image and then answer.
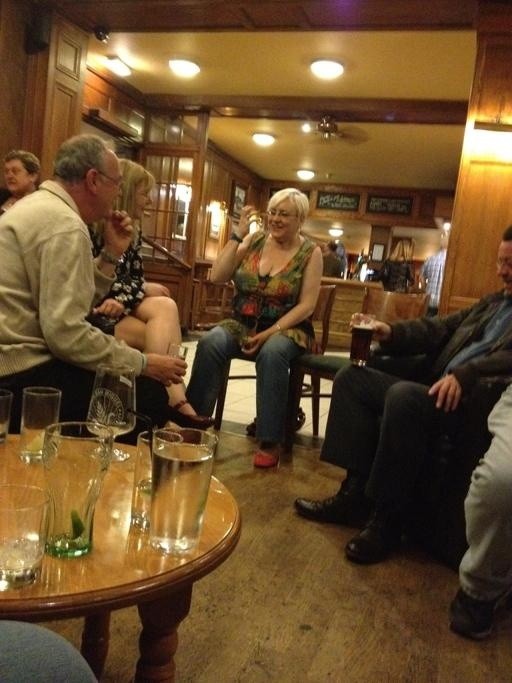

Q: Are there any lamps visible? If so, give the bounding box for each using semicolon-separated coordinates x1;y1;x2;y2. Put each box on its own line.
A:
309;59;346;82
251;131;276;148
297;169;315;182
329;228;343;236
168;60;199;79
106;56;132;80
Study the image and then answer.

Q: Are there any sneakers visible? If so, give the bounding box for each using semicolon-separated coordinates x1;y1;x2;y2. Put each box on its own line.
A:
447;586;507;641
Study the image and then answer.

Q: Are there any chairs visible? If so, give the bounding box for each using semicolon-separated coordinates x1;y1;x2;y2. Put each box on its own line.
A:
212;280;336;433
282;285;433;465
0;619;100;683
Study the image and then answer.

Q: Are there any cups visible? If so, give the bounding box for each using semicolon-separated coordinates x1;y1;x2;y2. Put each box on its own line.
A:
0;483;51;592
167;343;189;384
84;363;137;464
148;424;219;557
349;312;377;367
0;389;13;447
239;317;258;350
17;386;62;465
131;428;184;531
39;421;114;561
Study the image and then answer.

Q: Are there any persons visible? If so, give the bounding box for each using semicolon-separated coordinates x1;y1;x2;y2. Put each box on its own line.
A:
176;188;323;468
290;222;511;565
449;377;511;639
321;240;346;277
74;157;217;437
366;238;416;294
419;222;451;320
1;149;40;219
1;133;189;455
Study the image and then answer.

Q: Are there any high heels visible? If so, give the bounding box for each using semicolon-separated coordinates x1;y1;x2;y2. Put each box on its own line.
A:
165;397;215;429
251;444;281;467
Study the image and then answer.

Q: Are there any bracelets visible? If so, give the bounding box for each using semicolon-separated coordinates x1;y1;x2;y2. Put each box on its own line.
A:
274;320;282;333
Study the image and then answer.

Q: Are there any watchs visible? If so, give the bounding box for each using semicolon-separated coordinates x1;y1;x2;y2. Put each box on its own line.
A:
229;232;243;245
100;248;124;268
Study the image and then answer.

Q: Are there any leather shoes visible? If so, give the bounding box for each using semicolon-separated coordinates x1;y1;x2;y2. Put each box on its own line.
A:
344;503;409;563
294;471;370;525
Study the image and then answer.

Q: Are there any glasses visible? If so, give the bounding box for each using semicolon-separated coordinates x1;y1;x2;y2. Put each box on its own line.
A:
88;165;128;191
267;209;298;219
2;168;22;176
